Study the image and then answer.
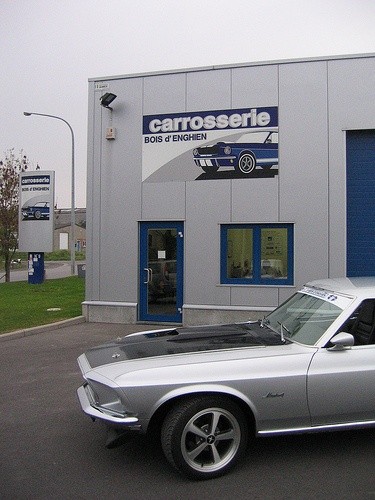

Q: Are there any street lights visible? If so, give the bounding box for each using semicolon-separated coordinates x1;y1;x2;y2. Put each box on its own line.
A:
23;111;76;275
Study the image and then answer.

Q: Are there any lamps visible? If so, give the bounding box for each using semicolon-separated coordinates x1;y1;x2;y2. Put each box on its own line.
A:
100;92;118;111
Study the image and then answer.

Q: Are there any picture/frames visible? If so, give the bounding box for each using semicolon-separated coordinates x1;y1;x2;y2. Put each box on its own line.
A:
228;240;233;257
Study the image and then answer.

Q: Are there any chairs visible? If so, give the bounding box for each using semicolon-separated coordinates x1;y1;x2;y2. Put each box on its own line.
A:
351;300;375;346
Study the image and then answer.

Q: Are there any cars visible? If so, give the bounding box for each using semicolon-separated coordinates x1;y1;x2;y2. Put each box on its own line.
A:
76;275;375;482
